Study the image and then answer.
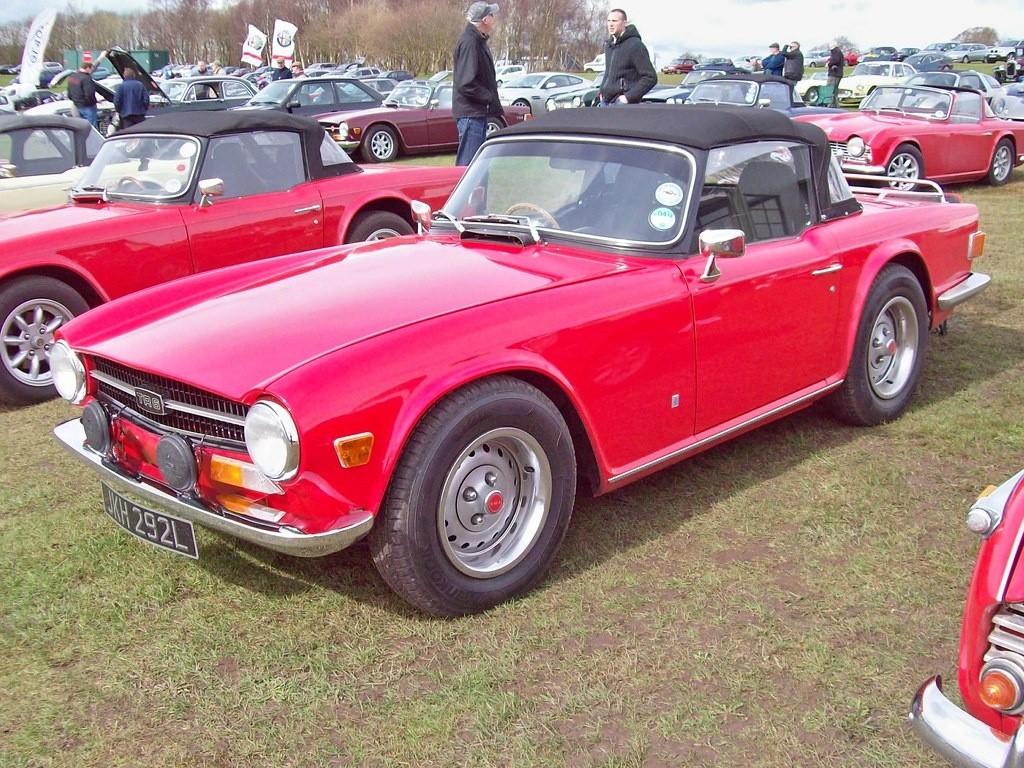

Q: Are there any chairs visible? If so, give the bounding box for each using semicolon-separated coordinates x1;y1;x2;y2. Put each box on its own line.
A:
212;141;249;177
274;143;305;192
733;162;809;243
318;90;332;104
301;92;314;105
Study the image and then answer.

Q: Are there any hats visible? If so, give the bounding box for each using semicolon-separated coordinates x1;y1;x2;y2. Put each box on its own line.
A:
212;60;222;67
467;1;499;23
276;57;284;63
769;43;779;47
291;61;302;69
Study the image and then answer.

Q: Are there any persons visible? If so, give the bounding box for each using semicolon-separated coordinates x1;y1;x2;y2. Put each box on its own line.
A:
452;1;504;214
762;41;804;85
825;41;843;107
191;59;225;76
68;50;149;130
272;57;307;81
598;9;658;107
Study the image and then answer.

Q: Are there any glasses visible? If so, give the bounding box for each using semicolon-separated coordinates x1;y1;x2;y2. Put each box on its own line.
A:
790;46;795;49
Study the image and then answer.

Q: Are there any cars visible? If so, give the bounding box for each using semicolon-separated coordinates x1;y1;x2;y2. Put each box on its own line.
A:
906;466;1024;768
0;39;1023;308
50;97;994;621
0;111;487;411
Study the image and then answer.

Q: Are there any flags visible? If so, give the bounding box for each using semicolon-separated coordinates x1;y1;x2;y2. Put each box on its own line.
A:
240;24;268;66
273;19;298;68
